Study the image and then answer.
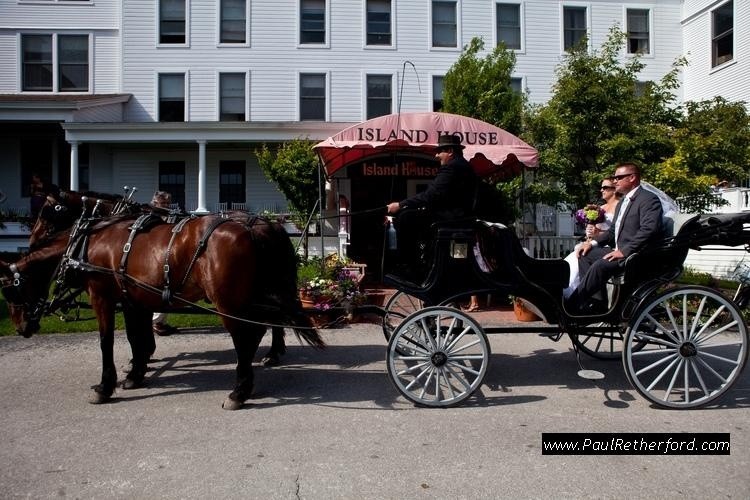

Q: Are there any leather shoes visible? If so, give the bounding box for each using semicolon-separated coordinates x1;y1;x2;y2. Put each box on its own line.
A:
576;301;609;316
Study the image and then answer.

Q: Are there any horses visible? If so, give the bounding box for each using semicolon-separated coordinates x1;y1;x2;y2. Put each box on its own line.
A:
29;187;330;367
0;209;272;412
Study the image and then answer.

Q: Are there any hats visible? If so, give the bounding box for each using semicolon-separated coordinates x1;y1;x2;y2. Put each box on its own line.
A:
433;134;465;151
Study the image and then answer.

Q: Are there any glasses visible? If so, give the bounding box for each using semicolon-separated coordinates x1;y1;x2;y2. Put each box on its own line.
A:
599;185;615;191
613;173;632;180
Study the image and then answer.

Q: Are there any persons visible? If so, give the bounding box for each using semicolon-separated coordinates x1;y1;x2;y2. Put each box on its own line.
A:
559;176;623;310
564;162;663;313
463;294;480;315
29;172;47;219
384;134;477;280
148;190;181;337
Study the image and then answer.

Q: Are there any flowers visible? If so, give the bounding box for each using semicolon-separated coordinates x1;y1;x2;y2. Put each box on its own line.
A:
310;268;361;322
576;202;607;227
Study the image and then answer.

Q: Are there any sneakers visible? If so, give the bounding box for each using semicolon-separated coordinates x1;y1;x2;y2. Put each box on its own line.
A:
152;322;179;337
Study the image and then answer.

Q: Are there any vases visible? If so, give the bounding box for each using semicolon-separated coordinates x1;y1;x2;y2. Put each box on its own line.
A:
512;300;539;321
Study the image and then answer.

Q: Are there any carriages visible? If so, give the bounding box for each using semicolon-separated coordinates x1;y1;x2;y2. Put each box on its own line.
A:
0;111;750;411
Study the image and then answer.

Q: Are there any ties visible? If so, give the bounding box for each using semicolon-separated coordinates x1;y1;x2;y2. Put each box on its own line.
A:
615;195;630;238
